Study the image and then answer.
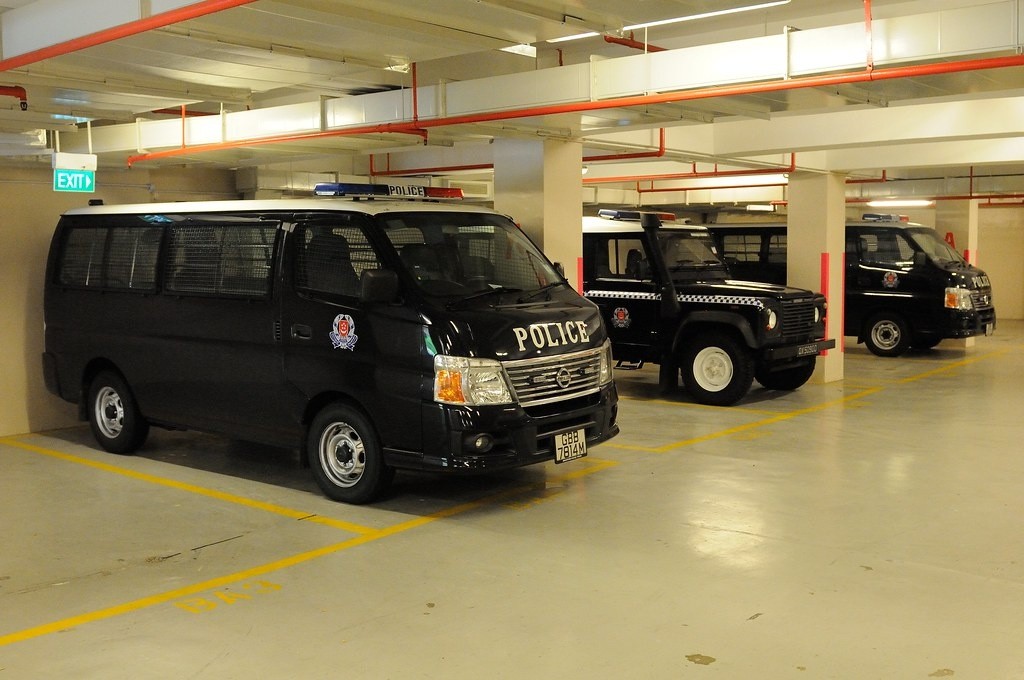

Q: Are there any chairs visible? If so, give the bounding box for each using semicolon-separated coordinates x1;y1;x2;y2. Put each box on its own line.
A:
400;243;448;295
876;239;896;262
861;238;869;260
305;233;359;294
624;249;645;278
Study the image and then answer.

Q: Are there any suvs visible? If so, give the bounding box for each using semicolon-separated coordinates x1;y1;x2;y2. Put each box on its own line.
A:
461;208;835;407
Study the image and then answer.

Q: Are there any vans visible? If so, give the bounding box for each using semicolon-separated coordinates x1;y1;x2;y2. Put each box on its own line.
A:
41;182;623;504
700;214;995;358
